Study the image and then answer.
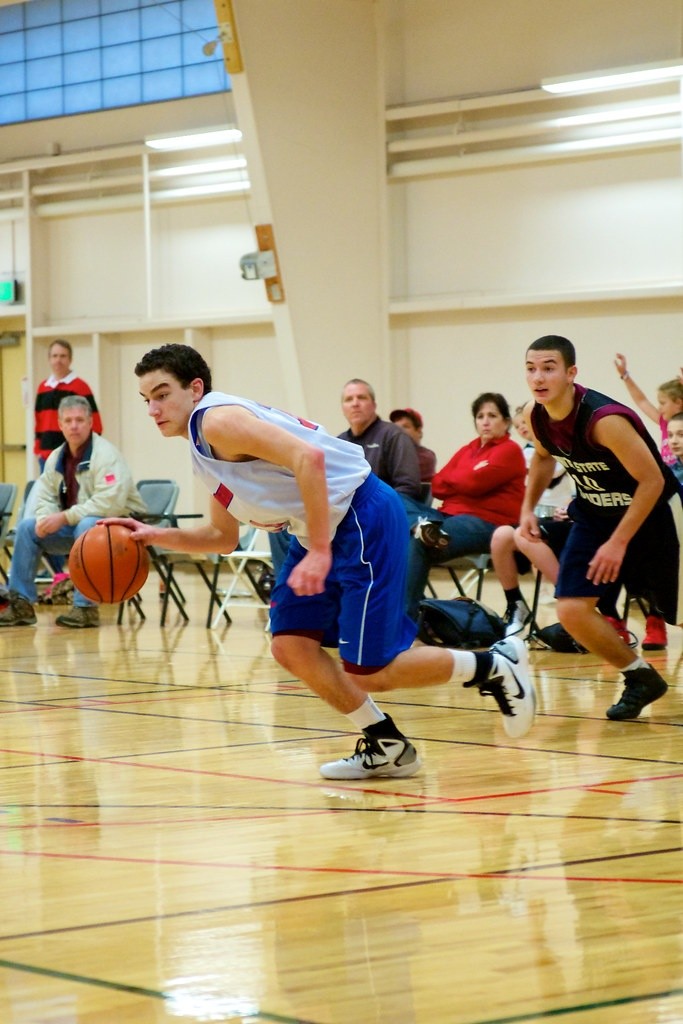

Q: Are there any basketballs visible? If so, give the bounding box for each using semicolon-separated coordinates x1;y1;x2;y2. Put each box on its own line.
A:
68;524;149;604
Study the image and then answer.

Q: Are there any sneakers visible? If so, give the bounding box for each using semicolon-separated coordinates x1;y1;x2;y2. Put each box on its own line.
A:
56;606;100;628
604;616;630;644
414;516;453;551
642;615;668;650
606;663;668;720
0;599;37;626
320;730;421;780
477;635;536;738
504;597;534;637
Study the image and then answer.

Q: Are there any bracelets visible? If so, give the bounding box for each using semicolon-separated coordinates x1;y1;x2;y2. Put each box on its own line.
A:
621;371;629;380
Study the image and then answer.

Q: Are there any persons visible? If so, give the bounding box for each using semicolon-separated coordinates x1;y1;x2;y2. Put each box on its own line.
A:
33;339;102;473
490;497;578;637
336;379;420;499
512;335;683;721
613;353;683;465
96;343;537;779
396;392;527;625
0;396;146;627
388;408;436;501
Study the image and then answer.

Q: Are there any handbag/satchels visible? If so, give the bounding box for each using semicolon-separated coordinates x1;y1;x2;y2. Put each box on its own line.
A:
417;597;507;648
535;622;589;654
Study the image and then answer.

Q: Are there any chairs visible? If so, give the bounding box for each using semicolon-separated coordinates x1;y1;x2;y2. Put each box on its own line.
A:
0;479;274;630
419;482;650;640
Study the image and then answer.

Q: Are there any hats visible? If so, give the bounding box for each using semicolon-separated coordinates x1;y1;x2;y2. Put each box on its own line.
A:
390;409;423;429
